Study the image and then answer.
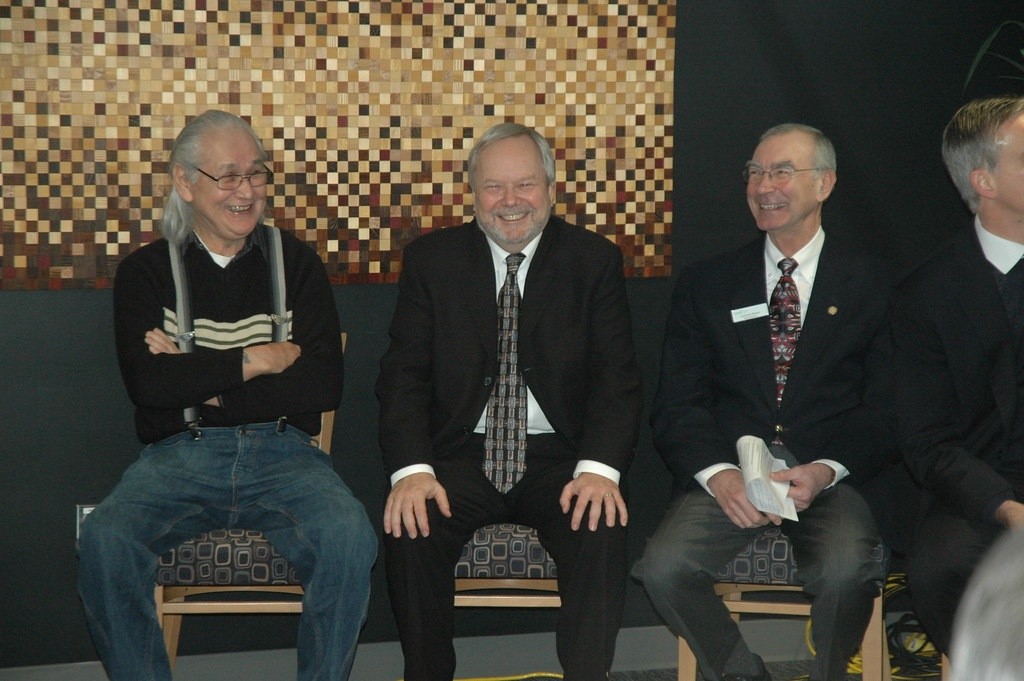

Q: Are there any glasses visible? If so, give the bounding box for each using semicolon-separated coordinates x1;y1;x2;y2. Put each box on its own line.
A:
192;164;273;190
742;166;819;184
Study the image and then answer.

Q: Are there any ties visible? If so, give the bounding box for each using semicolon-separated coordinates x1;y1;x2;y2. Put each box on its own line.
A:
768;258;801;447
483;252;526;496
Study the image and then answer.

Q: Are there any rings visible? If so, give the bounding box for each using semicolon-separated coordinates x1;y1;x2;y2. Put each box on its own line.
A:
604;493;614;498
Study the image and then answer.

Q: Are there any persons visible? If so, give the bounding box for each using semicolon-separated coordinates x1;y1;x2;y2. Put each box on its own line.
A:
374;122;640;681
79;110;379;681
629;122;885;681
861;95;1024;656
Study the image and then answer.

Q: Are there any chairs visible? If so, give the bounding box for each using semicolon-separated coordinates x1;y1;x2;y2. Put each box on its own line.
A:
143;332;348;673
677;523;891;681
454;522;563;608
